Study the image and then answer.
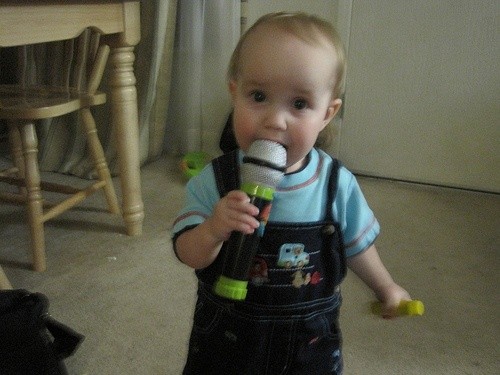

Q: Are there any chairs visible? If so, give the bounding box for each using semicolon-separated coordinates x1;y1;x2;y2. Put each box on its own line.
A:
1;27;120;272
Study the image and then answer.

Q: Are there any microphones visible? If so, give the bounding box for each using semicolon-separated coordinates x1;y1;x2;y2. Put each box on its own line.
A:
213;140;287;300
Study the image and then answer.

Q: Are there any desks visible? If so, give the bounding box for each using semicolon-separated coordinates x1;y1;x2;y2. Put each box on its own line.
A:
0;1;145;287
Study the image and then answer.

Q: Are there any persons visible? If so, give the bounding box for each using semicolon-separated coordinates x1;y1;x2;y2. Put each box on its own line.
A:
170;11;410;375
